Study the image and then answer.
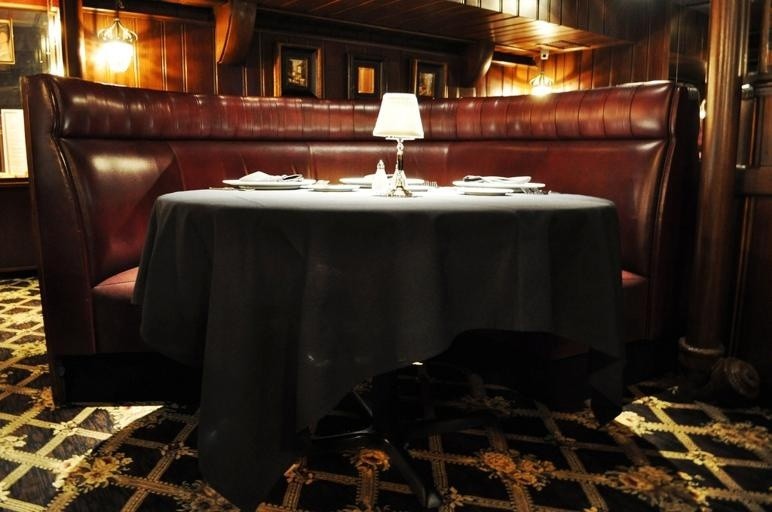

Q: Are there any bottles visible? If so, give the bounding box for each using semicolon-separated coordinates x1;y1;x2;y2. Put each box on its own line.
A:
372;161;389;197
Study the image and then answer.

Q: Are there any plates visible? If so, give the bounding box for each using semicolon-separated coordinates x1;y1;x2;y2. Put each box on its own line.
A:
452;180;546;195
222;177;424;191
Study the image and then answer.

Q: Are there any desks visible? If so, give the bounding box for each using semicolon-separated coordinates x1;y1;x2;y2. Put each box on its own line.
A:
159;182;620;511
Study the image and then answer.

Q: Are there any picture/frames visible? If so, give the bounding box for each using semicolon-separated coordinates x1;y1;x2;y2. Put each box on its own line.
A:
273;42;448;100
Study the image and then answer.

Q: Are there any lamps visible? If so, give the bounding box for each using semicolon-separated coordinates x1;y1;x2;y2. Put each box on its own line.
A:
372;92;426;197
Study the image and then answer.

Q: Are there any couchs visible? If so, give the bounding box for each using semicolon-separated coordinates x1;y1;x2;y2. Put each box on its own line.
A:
21;68;702;407
